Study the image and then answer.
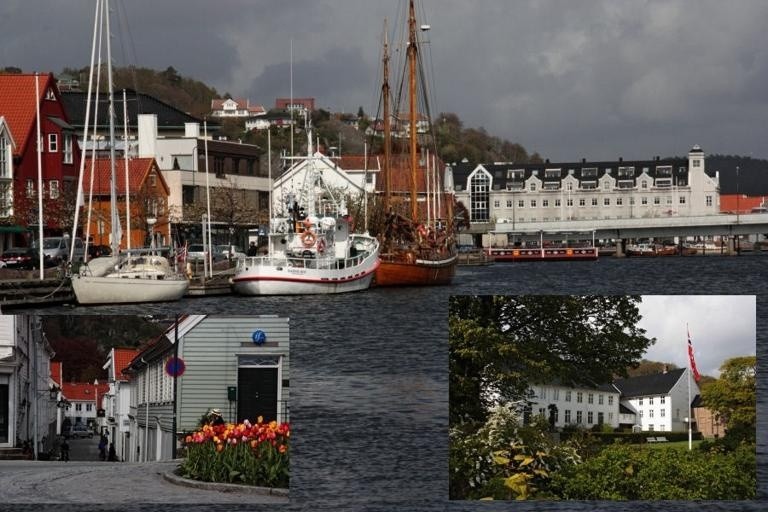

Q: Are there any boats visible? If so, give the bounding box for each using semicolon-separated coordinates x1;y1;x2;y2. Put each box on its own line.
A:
456;233;732;262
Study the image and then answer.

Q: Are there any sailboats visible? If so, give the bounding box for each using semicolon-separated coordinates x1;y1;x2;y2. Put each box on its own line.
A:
56;0;464;307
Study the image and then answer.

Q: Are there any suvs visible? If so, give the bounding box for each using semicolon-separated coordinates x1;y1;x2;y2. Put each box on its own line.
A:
62;425;95;440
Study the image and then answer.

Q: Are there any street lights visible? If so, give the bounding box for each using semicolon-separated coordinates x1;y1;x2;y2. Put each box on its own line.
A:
625;174;636;219
734;166;742;224
507;187;518;230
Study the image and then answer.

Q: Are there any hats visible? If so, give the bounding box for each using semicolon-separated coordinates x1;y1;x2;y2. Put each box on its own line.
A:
211;408;221;417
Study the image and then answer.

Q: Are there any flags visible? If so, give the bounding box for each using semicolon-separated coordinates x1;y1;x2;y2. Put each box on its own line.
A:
688;329;700;382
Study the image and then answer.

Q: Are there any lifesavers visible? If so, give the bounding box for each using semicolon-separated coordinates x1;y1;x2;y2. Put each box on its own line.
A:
301;232;316;247
318;239;325;251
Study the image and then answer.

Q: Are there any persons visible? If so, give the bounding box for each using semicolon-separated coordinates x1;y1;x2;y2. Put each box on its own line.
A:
209;408;225;426
246;241;257;257
93;428;119;461
59;440;69;462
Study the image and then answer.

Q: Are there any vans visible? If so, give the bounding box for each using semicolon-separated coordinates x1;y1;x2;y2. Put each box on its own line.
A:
750;207;768;215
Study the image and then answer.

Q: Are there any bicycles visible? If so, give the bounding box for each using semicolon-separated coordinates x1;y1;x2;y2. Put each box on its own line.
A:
60;449;72;462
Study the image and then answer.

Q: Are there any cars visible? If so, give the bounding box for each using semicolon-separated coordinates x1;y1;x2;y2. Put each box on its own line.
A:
1;233;114;272
716;210;734;216
